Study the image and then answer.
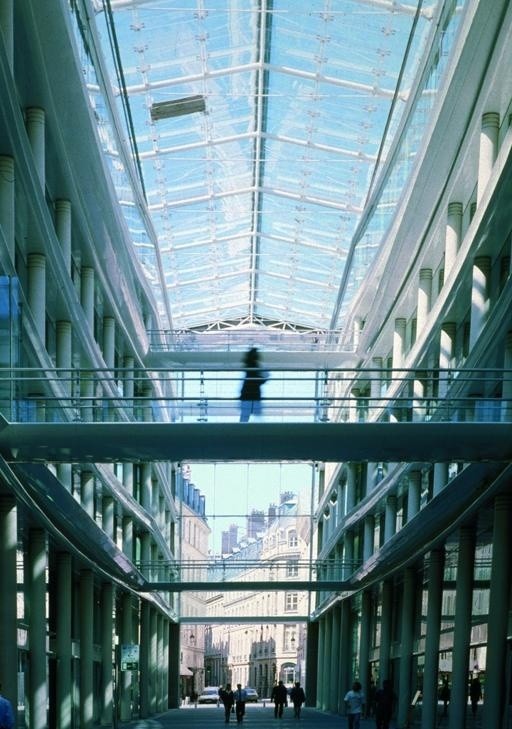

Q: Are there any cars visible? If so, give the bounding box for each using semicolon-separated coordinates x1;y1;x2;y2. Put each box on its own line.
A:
243;687;260;703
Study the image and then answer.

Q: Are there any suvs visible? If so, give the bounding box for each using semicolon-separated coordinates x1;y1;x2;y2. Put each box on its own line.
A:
196;684;221;704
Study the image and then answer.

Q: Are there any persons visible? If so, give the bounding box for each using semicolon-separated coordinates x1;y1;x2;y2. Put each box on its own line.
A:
343;682;367;728
233;684;249;725
221;684;233;723
218;684;224;703
270;680;287;719
470;677;483;721
365;681;376;719
371;679;396;727
291;680;305;718
0;682;16;729
239;345;266;421
